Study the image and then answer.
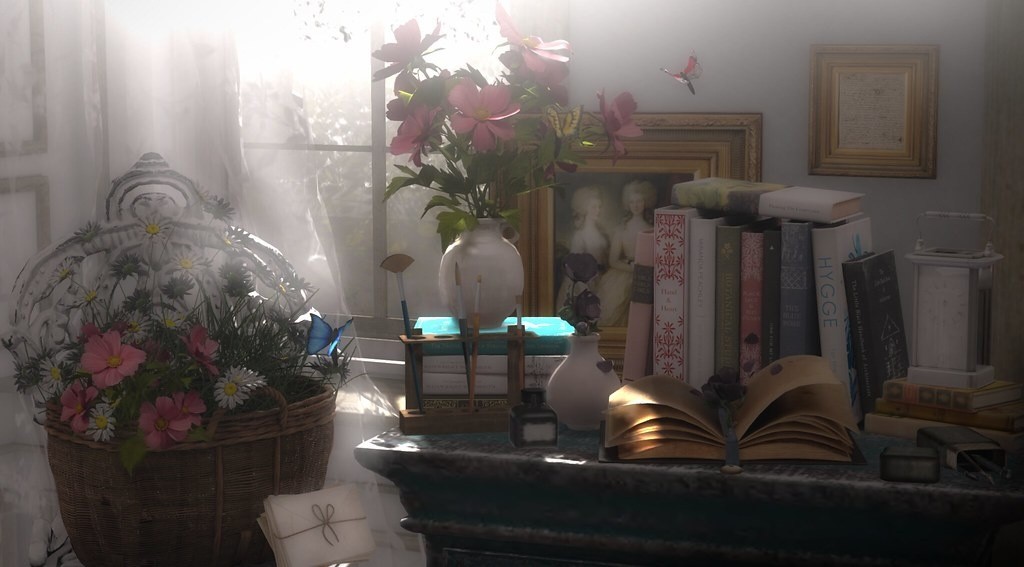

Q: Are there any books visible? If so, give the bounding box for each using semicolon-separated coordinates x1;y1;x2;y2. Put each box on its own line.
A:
621;176;1024;452
598;354;868;468
413;315;582;413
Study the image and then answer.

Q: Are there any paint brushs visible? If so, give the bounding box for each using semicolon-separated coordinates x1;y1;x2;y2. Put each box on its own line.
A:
455;261;470;394
515;294;523;388
470;274;482;412
380;253;423;414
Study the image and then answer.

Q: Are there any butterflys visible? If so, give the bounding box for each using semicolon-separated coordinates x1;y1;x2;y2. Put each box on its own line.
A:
665;50;702;96
308;313;353;356
547;104;582;139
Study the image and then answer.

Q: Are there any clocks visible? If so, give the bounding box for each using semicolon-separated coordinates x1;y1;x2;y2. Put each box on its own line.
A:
904;212;1004;390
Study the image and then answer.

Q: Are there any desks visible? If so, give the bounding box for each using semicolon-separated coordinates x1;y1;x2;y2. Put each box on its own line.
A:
354;433;1024;567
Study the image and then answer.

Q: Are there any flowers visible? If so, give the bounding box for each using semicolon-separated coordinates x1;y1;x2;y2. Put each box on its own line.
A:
2;191;370;473
549;246;604;338
376;48;645;241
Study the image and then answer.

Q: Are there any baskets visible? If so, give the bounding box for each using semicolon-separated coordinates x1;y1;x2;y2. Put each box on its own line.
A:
34;372;335;567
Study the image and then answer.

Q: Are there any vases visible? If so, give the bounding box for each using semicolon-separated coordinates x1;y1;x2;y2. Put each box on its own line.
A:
438;215;524;331
548;333;621;432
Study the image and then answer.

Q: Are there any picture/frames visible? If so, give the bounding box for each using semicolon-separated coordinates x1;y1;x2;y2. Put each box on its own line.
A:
517;110;763;360
809;43;940;180
